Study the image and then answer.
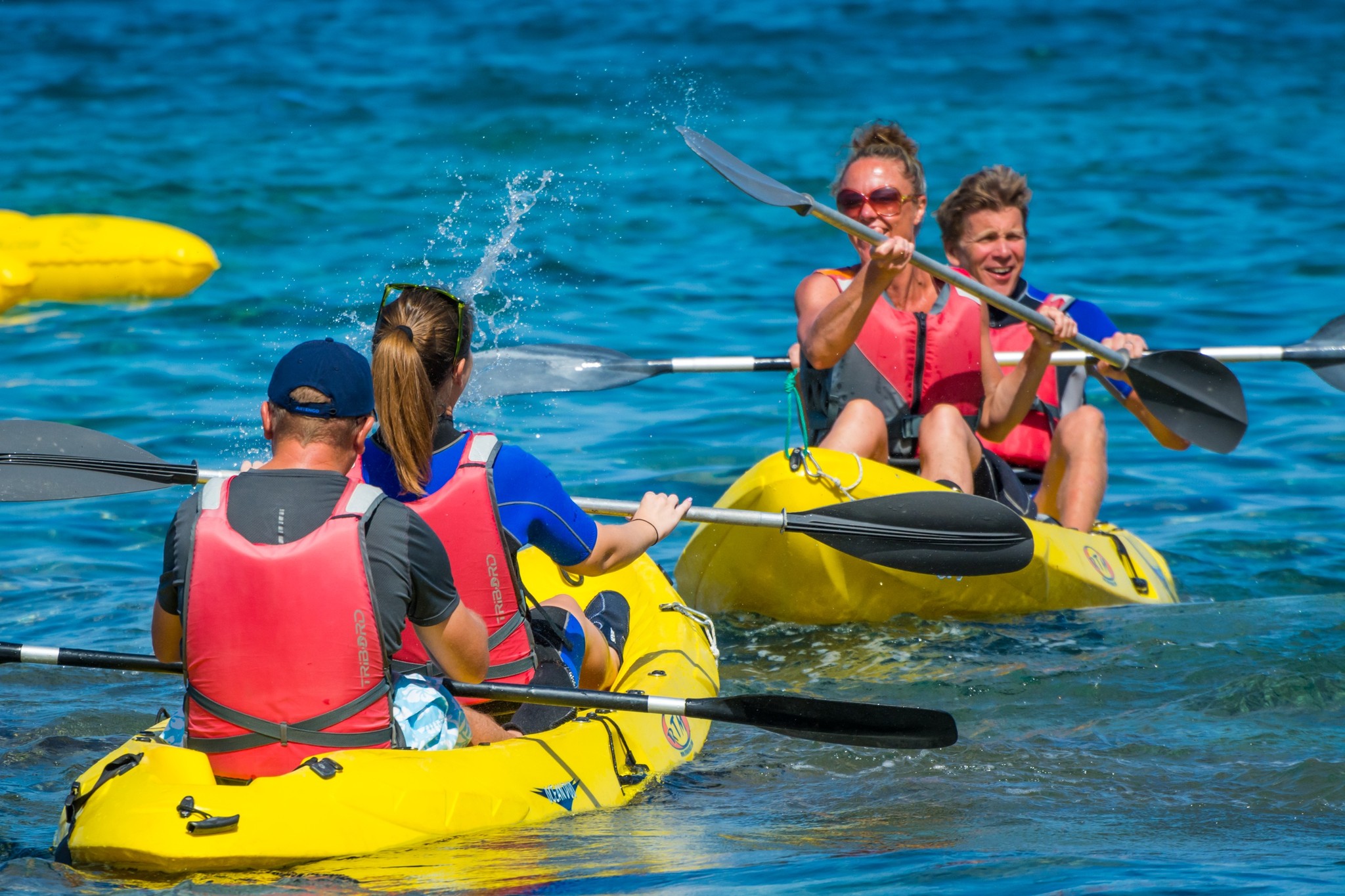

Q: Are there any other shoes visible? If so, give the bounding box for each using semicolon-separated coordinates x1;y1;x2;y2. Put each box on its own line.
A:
584;590;630;668
502;658;576;735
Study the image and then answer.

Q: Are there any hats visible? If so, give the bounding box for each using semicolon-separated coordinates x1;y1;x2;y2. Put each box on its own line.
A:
268;337;378;422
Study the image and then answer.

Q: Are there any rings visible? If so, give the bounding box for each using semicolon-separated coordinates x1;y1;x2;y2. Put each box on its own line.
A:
1124;341;1133;344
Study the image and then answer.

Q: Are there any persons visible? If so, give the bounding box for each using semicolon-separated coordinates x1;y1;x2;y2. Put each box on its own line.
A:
787;120;1191;538
151;281;695;787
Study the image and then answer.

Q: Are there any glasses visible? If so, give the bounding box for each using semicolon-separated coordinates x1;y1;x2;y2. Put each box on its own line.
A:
836;185;922;220
371;283;463;386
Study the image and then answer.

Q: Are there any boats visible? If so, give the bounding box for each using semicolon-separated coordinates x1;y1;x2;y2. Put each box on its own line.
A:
1;204;222;313
670;446;1181;627
50;546;721;873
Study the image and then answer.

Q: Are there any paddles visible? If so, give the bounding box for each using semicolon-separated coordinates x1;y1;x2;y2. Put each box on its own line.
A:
0;641;962;753
0;418;1036;579
456;314;1345;407
672;120;1249;457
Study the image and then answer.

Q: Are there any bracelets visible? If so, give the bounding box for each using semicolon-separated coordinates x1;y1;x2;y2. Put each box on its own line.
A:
630;519;659;546
